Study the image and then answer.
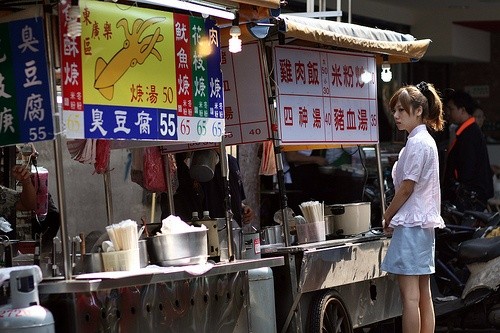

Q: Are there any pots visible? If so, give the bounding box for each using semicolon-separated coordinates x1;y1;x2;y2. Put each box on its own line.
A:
325;202;371;238
185;219;221;263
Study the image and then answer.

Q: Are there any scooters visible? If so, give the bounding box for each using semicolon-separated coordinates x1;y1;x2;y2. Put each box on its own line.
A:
430;198;499;327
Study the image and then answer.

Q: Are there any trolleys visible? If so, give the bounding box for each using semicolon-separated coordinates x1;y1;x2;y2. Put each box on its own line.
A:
0;1;284;332
159;15;431;333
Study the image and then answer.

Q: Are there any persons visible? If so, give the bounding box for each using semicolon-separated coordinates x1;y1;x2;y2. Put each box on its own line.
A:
193;148;252;228
442;88;500;226
384;81;445;333
18;147;61;251
0;148;36;219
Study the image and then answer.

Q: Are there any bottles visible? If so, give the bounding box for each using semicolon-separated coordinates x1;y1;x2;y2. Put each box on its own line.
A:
191;211;200;221
203;210;211;220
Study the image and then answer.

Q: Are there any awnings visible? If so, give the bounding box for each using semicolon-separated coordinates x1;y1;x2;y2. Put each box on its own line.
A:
284;15;430;63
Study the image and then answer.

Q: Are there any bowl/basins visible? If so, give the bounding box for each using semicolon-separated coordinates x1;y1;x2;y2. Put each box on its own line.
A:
143;229;209;267
82;252;104;273
262;224;284;244
138;239;149;268
